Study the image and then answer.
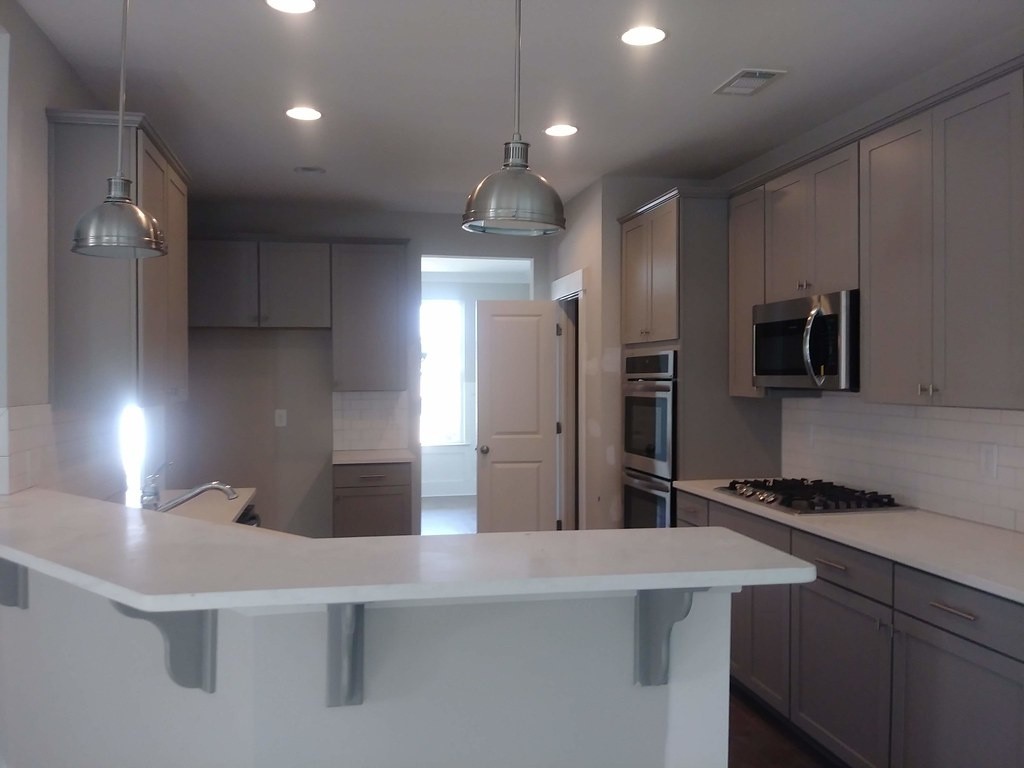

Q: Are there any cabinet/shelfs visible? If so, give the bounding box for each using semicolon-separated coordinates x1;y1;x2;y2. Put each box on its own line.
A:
189;240;408;393
618;64;1024;409
676;489;1024;768
48;125;187;406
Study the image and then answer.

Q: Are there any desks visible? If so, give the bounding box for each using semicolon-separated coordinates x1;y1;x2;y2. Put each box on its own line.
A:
0;487;816;768
332;449;413;537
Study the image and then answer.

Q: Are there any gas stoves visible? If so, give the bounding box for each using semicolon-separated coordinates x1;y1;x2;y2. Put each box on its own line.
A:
714;477;911;515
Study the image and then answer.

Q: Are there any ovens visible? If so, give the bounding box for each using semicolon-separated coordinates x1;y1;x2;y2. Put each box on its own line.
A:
621;348;675;481
621;467;676;528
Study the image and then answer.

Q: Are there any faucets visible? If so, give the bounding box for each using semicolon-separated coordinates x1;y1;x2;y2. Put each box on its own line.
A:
139;474;238;513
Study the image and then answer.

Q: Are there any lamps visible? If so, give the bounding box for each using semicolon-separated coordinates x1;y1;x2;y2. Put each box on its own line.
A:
461;1;566;236
72;0;166;259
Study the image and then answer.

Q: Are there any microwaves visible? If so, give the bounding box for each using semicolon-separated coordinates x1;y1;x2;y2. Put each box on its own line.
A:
752;290;859;391
